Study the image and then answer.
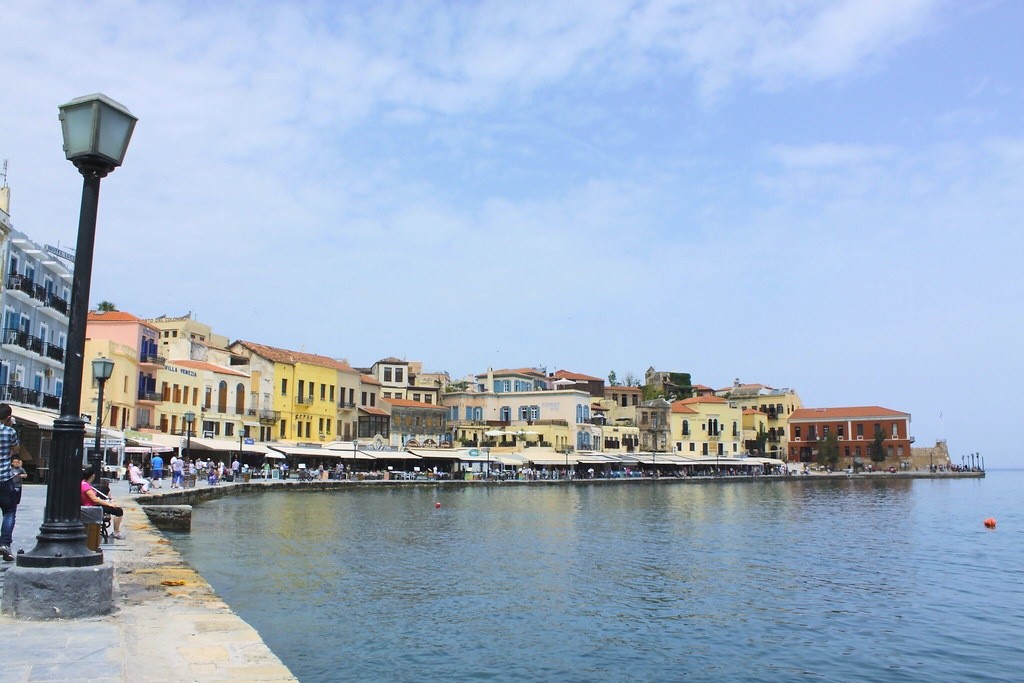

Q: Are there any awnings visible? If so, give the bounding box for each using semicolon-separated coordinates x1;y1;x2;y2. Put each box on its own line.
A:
269;447;459;460
457;449;676;465
189;436;272;453
7;404;122;438
676;452;786;466
125;432;187;447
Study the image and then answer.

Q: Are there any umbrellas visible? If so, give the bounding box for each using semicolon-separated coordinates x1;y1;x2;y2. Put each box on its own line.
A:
264;450;286;468
112;444;173;473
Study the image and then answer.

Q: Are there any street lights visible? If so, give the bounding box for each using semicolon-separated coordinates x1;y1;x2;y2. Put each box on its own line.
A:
564;449;570;478
352;438;358;478
486;447;491;478
183;410;196;476
962;451;983;472
238;428;246;479
651;449;657;477
715;453;720;476
14;93;141;568
89;356;115;500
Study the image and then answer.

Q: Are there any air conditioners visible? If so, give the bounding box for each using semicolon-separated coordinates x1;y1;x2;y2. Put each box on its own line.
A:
45;369;55;378
11;372;21;382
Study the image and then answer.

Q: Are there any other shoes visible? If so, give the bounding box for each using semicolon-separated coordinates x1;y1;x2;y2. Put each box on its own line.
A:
111;532;126;539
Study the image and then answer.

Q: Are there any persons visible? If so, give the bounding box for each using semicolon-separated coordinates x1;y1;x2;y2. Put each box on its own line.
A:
10;456;27;504
939;464;962;472
369;468;381;480
0;403;22;562
427;462;831;480
170;452;290;488
310;461;351;481
125;457;150;494
80;468;126;539
864;463;872;473
150;451;163;488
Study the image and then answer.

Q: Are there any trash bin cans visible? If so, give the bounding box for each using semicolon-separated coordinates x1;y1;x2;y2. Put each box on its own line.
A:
244;473;249;482
79;506;103;550
190;474;196;486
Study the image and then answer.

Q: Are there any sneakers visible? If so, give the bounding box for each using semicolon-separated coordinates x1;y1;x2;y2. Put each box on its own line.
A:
0;545;15;561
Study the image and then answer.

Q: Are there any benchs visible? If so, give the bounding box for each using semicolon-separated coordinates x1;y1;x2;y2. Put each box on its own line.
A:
296;476;314;482
127;470;144;493
100;514;111;544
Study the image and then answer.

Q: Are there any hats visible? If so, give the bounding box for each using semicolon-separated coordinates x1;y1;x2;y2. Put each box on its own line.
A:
154;452;160;455
207;458;210;460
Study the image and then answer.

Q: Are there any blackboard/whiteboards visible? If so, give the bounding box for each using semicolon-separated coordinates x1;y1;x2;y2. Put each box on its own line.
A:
272;469;279;479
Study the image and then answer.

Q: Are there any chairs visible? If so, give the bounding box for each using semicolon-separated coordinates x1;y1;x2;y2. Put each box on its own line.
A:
1;387;12;401
7;330;15;344
13;279;22;290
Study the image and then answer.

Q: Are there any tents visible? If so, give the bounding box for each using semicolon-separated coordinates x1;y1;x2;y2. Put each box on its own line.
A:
485;430;545;447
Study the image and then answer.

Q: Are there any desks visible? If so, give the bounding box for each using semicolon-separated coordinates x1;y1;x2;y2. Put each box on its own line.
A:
37;467;49;484
